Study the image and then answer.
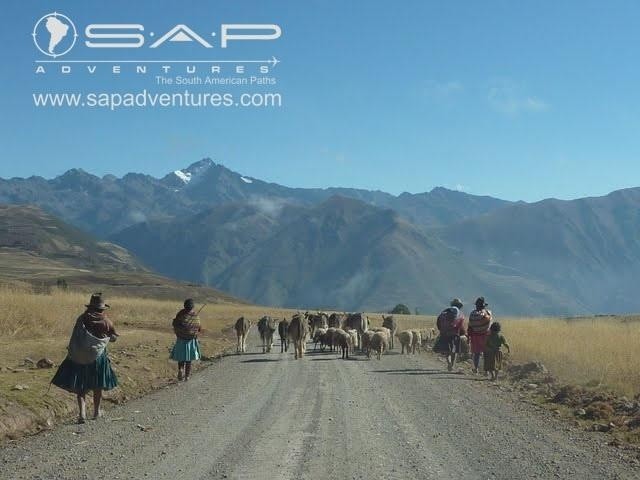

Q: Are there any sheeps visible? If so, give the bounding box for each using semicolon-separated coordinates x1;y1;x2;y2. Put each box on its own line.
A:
396;327;440;355
313;326;392;360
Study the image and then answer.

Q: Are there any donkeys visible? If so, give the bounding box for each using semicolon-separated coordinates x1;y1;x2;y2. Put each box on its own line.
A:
382;315;397;349
234;316;253;352
257;316;278;353
279;311;370;360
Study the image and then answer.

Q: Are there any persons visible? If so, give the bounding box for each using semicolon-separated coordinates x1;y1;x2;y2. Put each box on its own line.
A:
51;292;120;425
481;321;511;380
168;298;201;381
436;295;464;372
466;295;492;374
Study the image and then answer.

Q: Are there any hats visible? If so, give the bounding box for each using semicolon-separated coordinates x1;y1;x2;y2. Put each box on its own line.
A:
84;291;111;310
473;296;488;307
